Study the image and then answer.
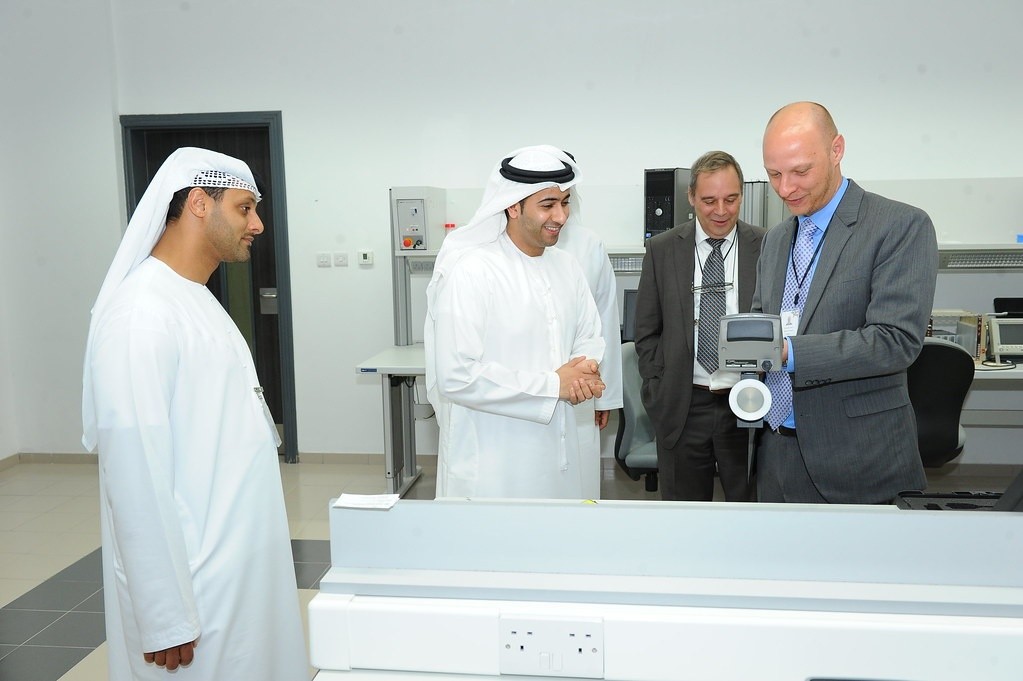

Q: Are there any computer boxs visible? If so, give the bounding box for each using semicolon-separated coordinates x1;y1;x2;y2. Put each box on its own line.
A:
644;167;693;247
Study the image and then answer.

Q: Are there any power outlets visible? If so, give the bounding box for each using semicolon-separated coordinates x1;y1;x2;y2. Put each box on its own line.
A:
499;614;604;680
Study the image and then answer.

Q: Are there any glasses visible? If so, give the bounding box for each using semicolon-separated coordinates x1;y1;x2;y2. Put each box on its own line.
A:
691;282;734;294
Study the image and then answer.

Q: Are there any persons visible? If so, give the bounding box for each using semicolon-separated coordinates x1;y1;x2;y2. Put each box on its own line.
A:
635;150;768;503
424;144;626;501
80;146;310;681
786;317;792;325
750;101;939;505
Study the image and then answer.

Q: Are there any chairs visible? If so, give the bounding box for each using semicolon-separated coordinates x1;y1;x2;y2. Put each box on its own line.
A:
906;337;975;469
614;339;719;491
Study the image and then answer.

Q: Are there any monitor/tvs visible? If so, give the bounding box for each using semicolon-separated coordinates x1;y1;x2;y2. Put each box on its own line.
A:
990;317;1023;356
623;290;637;340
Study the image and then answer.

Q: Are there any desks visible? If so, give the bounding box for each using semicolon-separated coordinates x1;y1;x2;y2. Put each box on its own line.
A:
973;360;1023;379
354;337;425;499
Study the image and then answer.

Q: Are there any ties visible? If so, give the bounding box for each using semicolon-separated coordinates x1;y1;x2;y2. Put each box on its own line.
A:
697;238;727;376
763;218;818;432
